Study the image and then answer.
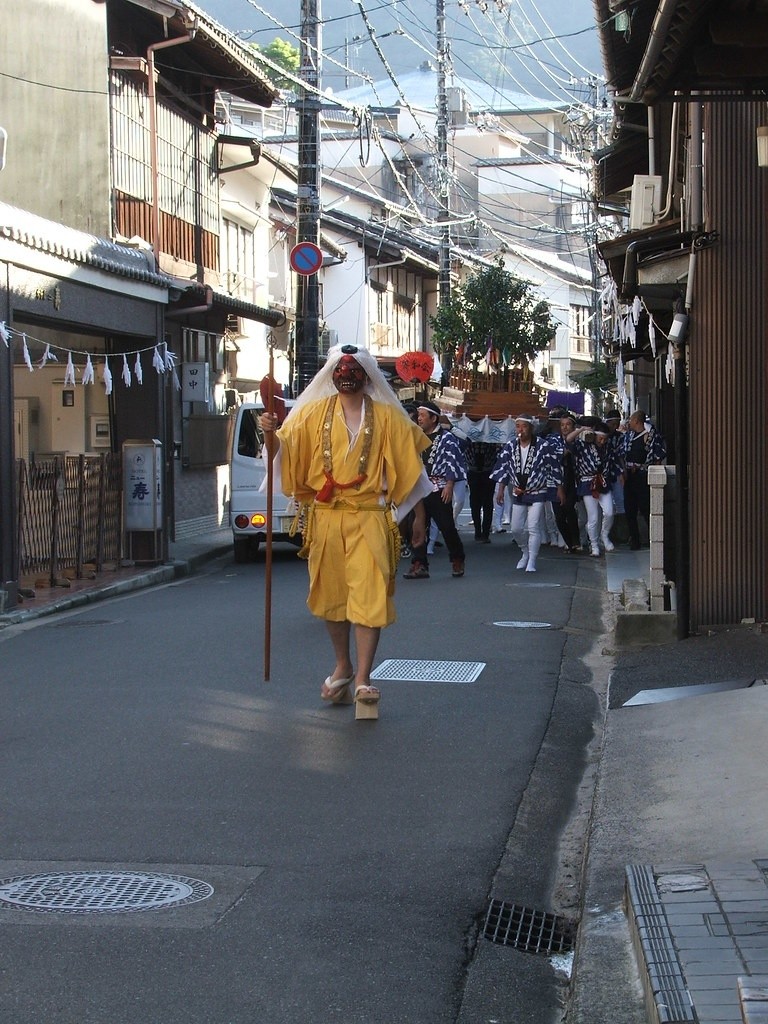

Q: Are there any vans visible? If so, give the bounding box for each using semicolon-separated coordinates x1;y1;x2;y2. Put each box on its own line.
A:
229;398;303;564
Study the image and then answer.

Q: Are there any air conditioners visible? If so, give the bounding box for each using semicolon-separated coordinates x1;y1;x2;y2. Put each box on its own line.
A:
630;174;662;229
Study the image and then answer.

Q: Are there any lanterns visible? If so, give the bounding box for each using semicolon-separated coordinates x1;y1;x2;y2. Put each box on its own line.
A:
395;350;434;385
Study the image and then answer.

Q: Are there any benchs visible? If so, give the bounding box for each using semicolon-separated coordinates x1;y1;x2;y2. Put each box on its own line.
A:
29;450;69;481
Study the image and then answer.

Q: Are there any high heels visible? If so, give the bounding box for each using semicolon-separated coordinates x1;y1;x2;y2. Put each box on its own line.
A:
354;685;380;720
321;672;355;704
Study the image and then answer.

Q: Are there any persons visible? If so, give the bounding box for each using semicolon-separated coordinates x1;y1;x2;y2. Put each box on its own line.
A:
400;401;666;580
261;342;434;721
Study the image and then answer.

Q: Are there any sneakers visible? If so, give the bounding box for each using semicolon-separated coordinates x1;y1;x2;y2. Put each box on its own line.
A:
452;558;465;577
403;562;430;579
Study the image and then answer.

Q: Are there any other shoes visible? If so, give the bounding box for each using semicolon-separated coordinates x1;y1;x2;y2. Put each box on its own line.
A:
400;518;641;572
